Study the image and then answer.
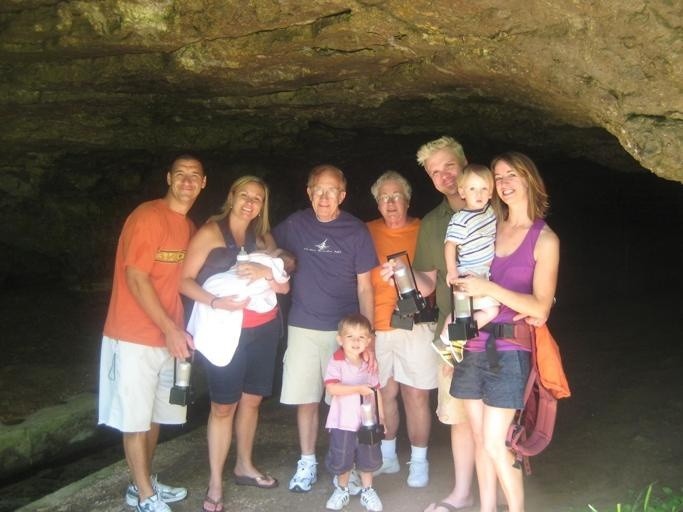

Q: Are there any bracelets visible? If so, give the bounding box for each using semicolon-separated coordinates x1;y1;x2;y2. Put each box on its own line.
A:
370;327;376;337
211;295;221;310
265;277;273;281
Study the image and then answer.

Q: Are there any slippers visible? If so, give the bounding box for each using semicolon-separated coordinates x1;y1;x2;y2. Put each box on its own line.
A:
423;501;475;512
234;472;278;488
202;487;224;512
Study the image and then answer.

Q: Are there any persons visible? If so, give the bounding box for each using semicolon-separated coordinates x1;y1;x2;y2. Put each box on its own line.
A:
273;167;379;495
380;135;556;511
96;156;207;512
363;171;437;488
449;152;561;511
431;164;508;369
179;175;291;512
270;246;298;276
323;314;387;511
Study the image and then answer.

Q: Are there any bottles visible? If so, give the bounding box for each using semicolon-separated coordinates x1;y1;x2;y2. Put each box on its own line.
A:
235;247;250;266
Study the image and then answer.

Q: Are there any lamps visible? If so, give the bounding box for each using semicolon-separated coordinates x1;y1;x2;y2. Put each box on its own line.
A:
444;272;482;343
355;383;386;448
389;300;414;333
384;248;426;319
168;339;197;409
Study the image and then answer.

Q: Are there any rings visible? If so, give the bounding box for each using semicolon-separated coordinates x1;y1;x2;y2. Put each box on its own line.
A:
460;286;464;292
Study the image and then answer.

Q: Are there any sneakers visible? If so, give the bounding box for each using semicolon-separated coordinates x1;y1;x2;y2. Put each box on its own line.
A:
360;486;382;511
326;485;349;510
125;472;187;506
137;492;172;512
288;459;317;492
333;469;362;495
407;457;429;487
372;453;400;475
